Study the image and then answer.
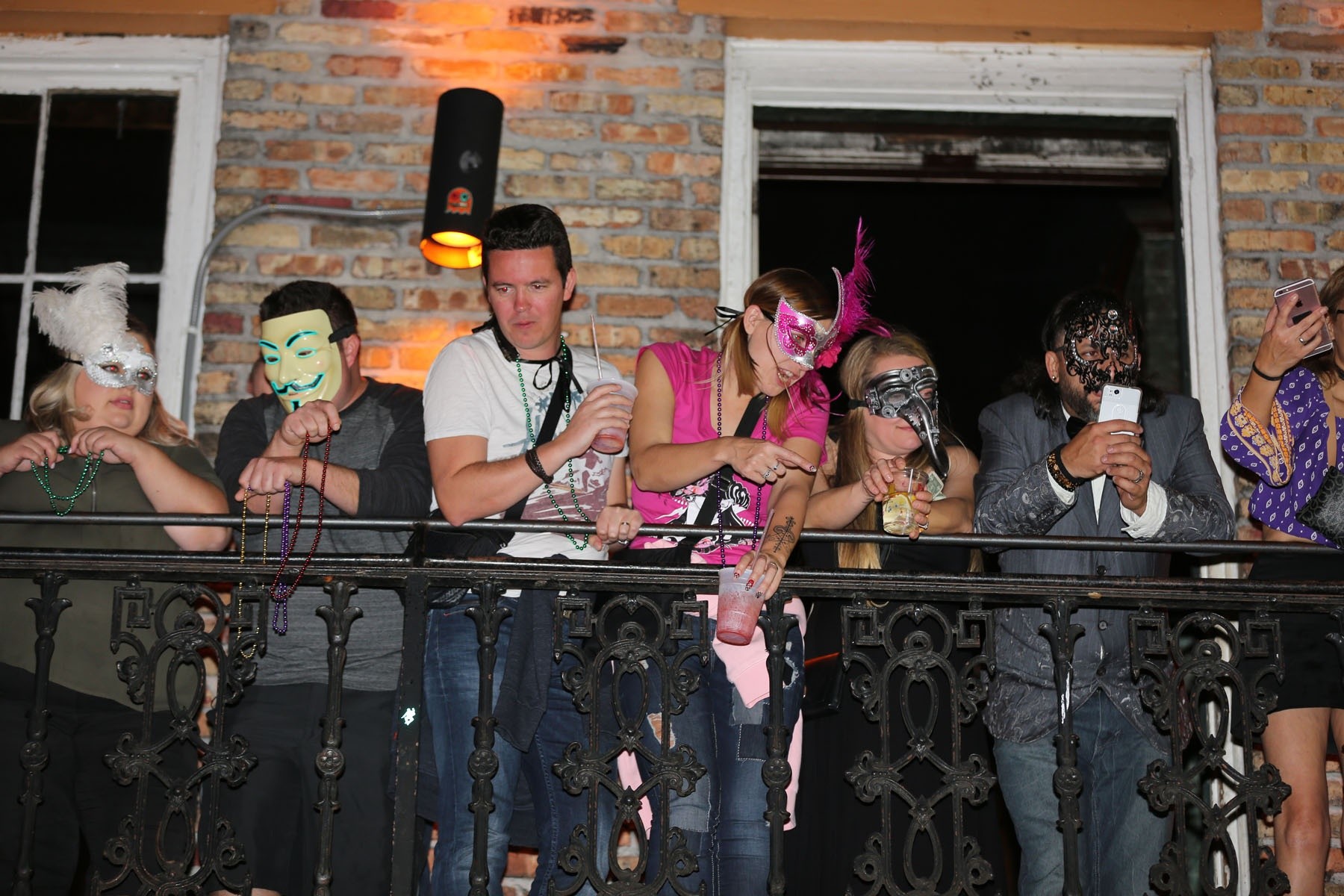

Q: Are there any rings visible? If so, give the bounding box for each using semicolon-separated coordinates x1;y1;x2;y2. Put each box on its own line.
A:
620;521;630;526
762;467;771;479
1131;469;1144;484
770;562;779;572
1297;336;1307;345
770;459;779;470
617;540;629;546
917;517;929;529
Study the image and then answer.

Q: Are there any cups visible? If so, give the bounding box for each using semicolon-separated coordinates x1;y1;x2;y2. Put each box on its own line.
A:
586;377;639;455
716;567;766;646
881;465;930;536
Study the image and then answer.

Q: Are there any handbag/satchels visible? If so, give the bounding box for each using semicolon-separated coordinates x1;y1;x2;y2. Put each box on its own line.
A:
399;510;508;604
1295;465;1344;549
584;543;691;656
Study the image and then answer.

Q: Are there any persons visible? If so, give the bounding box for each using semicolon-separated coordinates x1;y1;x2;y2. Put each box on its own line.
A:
795;331;1007;896
423;203;643;896
215;279;434;896
0;262;233;896
972;288;1236;896
1219;264;1344;895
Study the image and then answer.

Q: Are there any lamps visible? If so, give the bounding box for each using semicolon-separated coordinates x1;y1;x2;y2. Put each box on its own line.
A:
417;88;505;272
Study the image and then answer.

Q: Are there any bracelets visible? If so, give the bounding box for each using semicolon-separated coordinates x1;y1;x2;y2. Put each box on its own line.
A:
1252;361;1285;381
525;447;554;484
1047;448;1080;492
1055;442;1085;483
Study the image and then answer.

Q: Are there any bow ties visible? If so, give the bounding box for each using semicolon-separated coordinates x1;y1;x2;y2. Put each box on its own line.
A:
1065;416;1087;440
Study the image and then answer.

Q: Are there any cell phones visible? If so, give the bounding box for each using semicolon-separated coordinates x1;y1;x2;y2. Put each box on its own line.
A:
1274;278;1333;359
1098;383;1142;436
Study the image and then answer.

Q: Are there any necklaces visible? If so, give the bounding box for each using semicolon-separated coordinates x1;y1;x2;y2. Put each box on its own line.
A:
716;350;769;568
29;444;104;516
516;334;592;551
237;424;332;659
